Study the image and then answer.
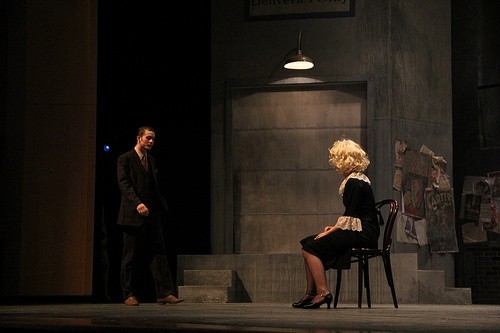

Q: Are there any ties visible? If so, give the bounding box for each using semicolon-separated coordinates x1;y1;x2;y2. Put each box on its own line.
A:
142;155;147;169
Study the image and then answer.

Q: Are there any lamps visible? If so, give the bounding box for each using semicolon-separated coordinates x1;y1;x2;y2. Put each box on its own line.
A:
283;26;314;70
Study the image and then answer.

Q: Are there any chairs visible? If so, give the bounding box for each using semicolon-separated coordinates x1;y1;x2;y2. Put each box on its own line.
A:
333;198;399;308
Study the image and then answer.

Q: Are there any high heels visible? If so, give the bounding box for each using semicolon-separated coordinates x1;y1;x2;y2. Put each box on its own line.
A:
292;290;317;308
304;292;333;309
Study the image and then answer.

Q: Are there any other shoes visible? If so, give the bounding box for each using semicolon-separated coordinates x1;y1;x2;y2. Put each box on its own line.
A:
157;295;185;304
124;295;138;306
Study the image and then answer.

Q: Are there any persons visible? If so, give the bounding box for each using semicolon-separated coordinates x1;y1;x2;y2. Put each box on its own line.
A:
116;127;185;306
290;139;381;308
